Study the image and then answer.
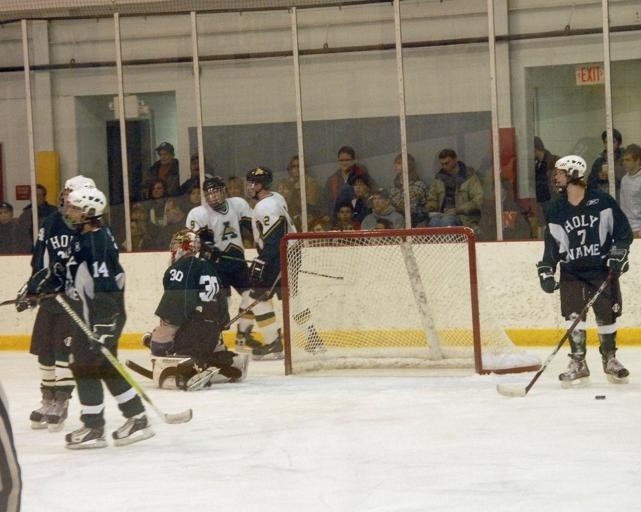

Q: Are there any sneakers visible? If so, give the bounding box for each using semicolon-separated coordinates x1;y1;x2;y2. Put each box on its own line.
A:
112;411;152;440
235;323;252;345
143;333;152;346
559;352;590;381
47;391;69;424
65;425;104;444
252;328;283;355
602;352;629;377
30;392;53;421
305;324;321;352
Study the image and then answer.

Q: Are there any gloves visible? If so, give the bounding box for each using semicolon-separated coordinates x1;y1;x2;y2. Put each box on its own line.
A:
606;248;629;279
536;261;559;293
203;240;220;261
15;284;37;312
88;321;121;357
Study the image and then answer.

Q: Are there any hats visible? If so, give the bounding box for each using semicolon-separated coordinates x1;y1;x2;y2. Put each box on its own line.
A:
64;175;95;218
246;167;273;199
155;142;174;155
67;186;107;224
170;230;201;265
551;155;587;192
203;176;225;210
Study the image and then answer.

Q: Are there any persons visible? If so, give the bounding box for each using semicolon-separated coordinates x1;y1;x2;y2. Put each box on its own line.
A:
19;184;57;228
534;135;562;222
351;175;372;220
537;155;634;389
330;202;360;230
360;188;405;230
156;197;190;250
246;167;327;360
276;180;300;217
292;204;323;232
16;189;155;450
145;179;172;239
425;148;483;227
620;144;641;238
121;221;144;253
131;203;146;221
287;155;320;208
151;228;226;356
145;142;179;194
186;177;261;352
181;184;201;213
0;383;22;512
317;147;368;223
487;176;531;241
388;153;429;227
30;176;96;432
174;155;212;196
0;202;32;252
587;129;626;207
225;177;254;208
